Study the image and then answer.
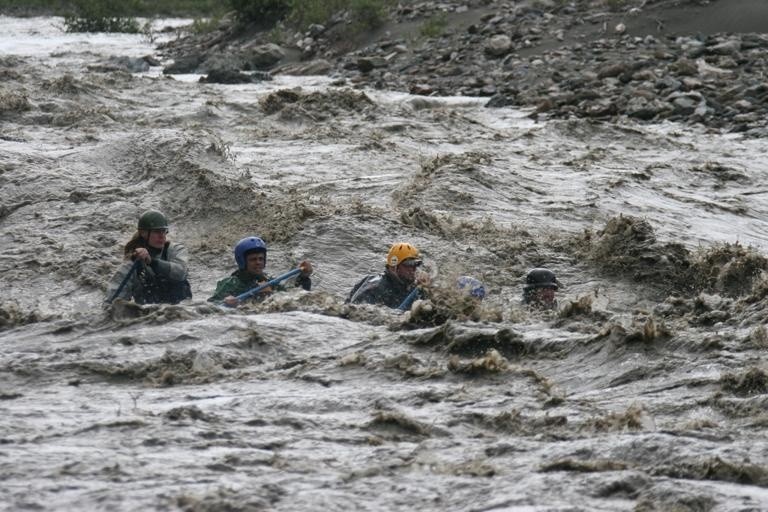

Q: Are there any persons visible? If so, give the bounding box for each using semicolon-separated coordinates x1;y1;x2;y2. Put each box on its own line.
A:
453;276;487;318
521;267;563;311
207;235;313;307
349;241;433;310
101;208;194;311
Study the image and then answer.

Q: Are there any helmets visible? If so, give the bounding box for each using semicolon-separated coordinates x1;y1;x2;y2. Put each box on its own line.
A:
455;276;485;298
234;237;266;270
387;243;419;268
138;211;168;231
522;269;558;292
